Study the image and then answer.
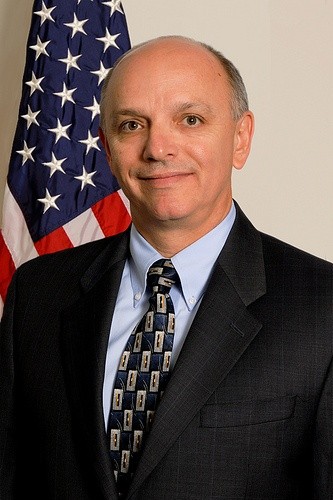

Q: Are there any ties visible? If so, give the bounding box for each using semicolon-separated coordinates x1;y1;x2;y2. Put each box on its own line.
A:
105;259;187;495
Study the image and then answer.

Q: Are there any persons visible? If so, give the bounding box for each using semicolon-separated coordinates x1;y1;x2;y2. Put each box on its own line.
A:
1;35;332;499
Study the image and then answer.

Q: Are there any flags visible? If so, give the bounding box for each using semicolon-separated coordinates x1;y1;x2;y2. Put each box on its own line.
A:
1;0;133;306
